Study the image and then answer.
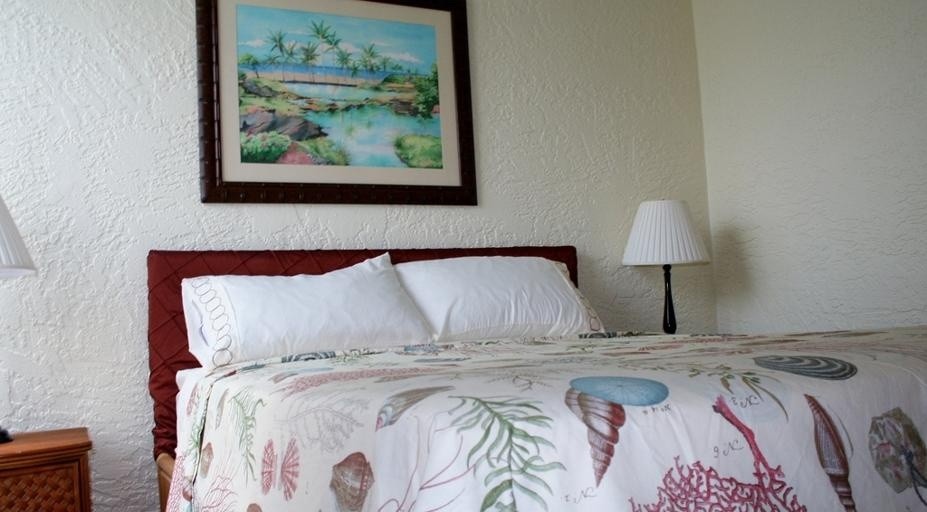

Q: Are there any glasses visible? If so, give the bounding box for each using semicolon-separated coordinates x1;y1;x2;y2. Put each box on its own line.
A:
622;198;710;334
0;195;38;442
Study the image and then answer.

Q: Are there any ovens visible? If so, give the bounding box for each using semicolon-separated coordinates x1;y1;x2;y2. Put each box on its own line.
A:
146;246;927;512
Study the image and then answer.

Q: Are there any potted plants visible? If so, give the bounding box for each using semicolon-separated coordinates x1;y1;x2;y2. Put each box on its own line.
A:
194;0;478;207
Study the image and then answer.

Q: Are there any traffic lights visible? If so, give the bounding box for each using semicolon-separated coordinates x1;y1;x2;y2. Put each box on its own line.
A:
395;256;608;342
181;252;432;369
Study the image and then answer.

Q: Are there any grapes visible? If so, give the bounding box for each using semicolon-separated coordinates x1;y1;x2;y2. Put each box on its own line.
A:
0;426;94;512
